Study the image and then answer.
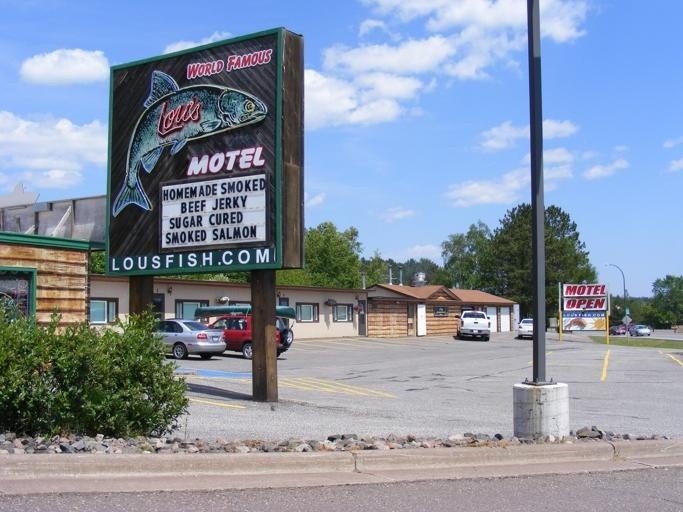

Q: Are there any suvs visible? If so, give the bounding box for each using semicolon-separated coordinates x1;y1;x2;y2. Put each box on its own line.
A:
207;314;293;359
454;310;490;341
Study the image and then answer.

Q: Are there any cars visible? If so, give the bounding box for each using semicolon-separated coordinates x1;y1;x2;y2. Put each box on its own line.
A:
609;324;651;337
517;318;533;339
153;319;226;359
